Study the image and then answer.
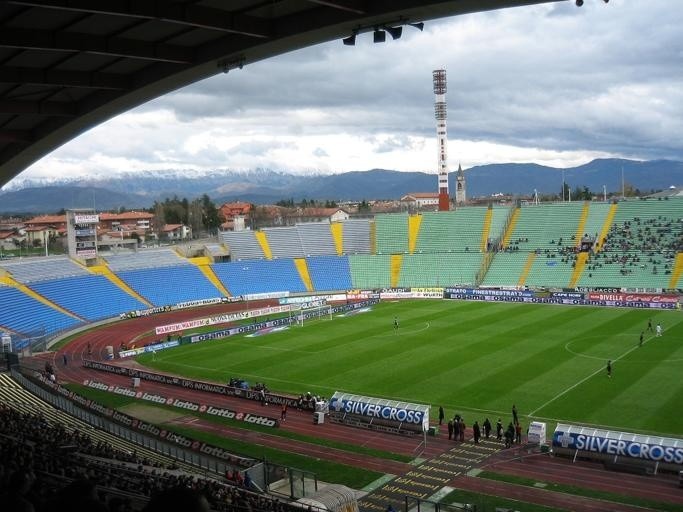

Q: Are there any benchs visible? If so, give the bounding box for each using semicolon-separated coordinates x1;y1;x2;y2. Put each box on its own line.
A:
0;372;218;481
496;195;682;298
242;203;494;295
1;231;242;343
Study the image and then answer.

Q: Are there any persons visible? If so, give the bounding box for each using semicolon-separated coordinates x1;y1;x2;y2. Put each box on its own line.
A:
437;403;521;449
63;354;67;365
484;214;682;277
638;331;644;346
392;316;399;334
87;341;136;360
655;323;662;337
230;377;327;402
177;331;182;344
646;317;652;331
167;333;170;342
606;360;612;379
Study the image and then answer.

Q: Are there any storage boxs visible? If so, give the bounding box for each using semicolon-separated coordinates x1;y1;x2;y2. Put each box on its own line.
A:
0;372;218;481
496;195;682;298
